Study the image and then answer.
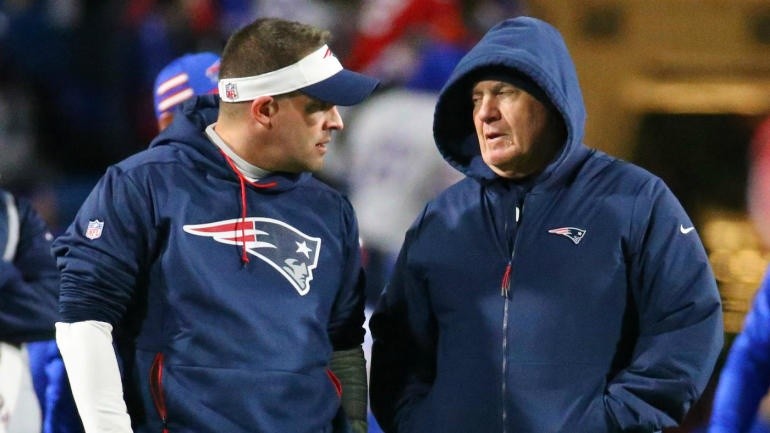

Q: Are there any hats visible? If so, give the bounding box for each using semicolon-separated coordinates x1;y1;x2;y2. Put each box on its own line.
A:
218;44;380;104
154;52;221;118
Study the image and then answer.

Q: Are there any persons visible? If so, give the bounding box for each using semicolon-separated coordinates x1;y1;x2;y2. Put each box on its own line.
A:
152;47;223;139
710;266;770;433
21;179;94;432
0;175;61;433
56;19;385;433
370;14;720;433
